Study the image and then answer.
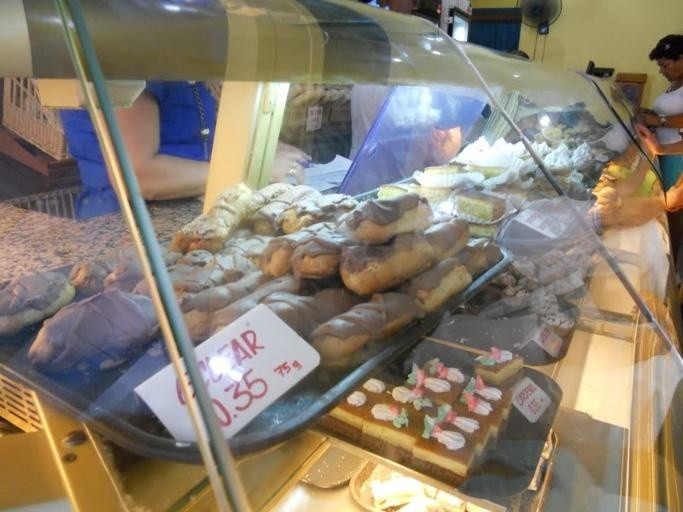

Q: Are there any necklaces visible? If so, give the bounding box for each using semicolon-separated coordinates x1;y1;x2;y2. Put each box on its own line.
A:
191;81;210;161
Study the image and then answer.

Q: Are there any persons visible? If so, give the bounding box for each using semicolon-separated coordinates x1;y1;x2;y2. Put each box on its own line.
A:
55;81;315;219
345;84;402;160
632;33;682;191
635;124;683;215
659;117;667;127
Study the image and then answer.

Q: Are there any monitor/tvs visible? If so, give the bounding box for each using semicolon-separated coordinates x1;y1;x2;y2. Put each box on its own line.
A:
450;6;471;42
585;60;595;75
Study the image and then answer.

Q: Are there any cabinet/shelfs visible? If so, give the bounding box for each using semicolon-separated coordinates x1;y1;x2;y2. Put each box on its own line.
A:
0;0;683;512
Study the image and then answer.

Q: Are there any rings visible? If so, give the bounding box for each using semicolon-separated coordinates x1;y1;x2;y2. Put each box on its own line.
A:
288;165;299;177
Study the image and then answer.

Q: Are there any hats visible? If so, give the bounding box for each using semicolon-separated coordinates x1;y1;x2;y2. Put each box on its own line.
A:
649;34;683;60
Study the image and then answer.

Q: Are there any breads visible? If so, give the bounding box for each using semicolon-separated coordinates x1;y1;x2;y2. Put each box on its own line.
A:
370;476;489;512
0;162;506;375
491;245;591;338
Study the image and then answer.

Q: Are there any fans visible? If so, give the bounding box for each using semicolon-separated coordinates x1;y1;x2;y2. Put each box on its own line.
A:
516;0;562;34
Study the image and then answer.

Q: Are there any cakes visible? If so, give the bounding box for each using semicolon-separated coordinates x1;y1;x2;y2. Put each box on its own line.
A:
527;140;595;196
326;345;524;488
